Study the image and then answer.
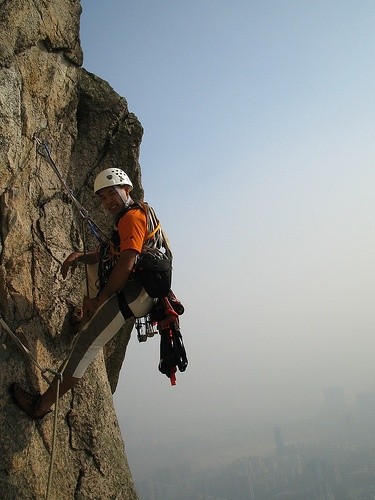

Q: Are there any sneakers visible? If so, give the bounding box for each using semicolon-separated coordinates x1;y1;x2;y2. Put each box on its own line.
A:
11;381;47;420
68;315;83;344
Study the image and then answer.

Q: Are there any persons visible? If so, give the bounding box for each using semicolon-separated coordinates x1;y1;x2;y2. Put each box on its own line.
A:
10;168;174;420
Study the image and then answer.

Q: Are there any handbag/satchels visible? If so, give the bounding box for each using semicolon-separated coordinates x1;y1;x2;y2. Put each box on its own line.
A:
139;254;172;298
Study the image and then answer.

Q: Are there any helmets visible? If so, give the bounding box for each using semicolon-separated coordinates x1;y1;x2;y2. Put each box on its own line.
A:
93;167;134;197
